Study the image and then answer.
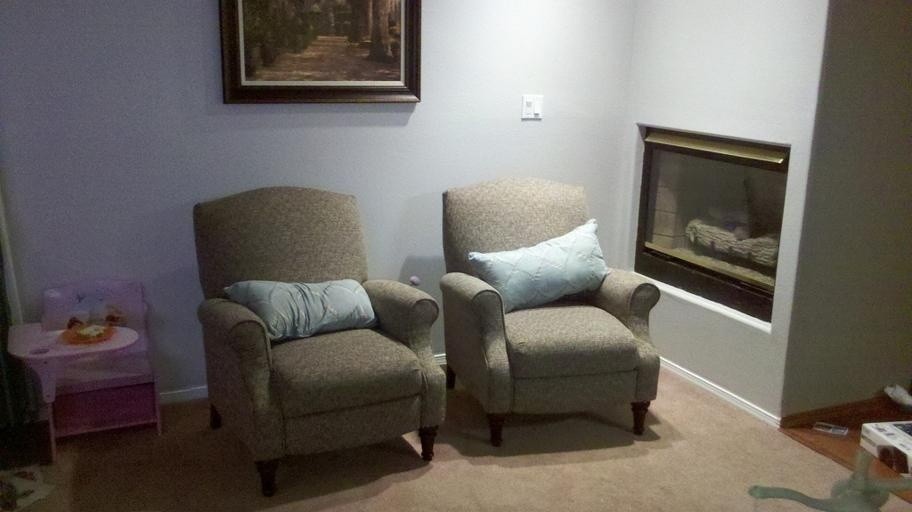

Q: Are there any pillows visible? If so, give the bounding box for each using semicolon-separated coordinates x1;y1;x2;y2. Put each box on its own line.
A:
468;219;611;314
223;279;375;342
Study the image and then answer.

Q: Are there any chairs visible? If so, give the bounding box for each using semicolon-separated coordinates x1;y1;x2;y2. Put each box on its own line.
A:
440;176;660;446
6;280;161;463
193;187;446;496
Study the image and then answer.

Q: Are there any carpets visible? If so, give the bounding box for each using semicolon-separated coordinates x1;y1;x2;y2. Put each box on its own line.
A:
20;362;908;512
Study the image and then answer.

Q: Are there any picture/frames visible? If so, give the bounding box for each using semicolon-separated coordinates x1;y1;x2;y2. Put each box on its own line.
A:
220;0;421;104
